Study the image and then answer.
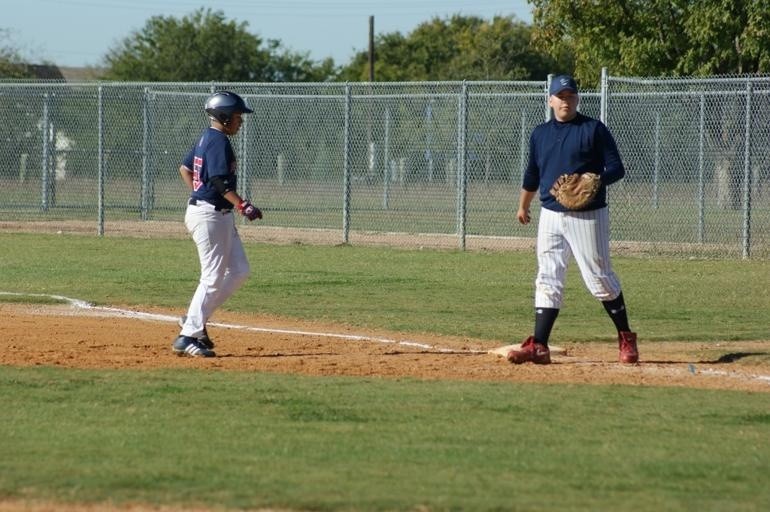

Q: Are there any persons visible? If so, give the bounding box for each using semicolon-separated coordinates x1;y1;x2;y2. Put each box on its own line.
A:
507;75;639;364
171;90;262;358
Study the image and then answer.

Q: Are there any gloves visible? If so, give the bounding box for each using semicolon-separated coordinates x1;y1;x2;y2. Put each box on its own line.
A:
234;199;262;221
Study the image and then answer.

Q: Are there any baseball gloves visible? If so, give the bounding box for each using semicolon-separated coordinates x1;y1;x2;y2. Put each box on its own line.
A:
550;172;602;210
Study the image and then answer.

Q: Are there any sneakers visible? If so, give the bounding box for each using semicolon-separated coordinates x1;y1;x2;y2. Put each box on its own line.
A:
172;315;216;358
618;331;639;363
507;336;550;364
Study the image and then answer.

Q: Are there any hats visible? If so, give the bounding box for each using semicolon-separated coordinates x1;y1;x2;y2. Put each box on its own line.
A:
549;76;578;96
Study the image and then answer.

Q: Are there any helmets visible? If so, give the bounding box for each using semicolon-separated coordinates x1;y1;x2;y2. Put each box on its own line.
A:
204;91;254;127
